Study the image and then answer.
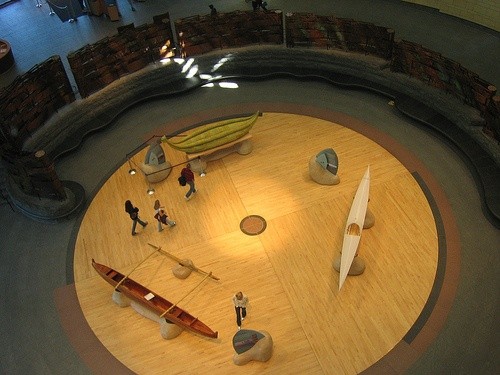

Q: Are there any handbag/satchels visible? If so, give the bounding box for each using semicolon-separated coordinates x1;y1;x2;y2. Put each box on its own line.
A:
131;212;137;219
178;177;186;186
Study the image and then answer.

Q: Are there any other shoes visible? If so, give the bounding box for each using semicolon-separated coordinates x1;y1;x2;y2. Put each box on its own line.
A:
162;228;164;230
242;317;244;320
184;196;189;201
132;232;136;235
238;326;240;330
192;190;197;194
174;222;176;225
143;222;147;226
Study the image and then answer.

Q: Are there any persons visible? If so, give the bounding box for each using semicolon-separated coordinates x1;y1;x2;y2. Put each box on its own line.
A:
233;292;248;331
153;200;176;232
252;0;266;11
208;3;216;13
125;200;148;236
181;163;197;200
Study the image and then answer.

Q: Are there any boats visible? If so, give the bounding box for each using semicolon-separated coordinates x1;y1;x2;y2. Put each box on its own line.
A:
337;165;373;289
90;258;219;339
162;110;263;155
